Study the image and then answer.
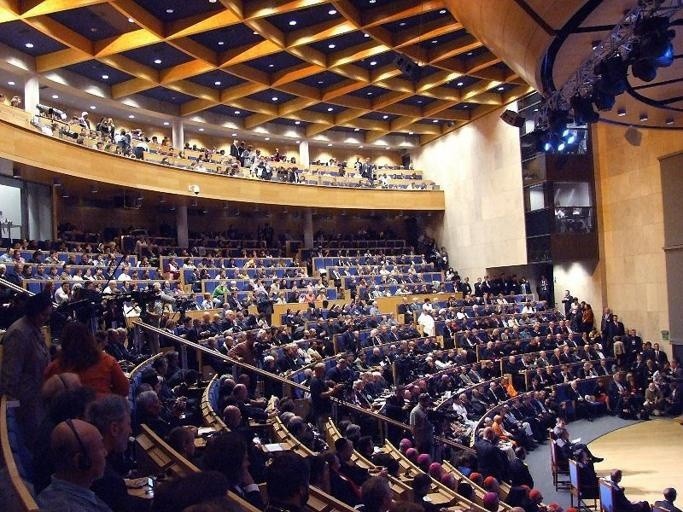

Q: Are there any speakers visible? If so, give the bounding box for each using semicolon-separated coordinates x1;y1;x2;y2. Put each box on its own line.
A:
394;55;422;83
499;109;525;127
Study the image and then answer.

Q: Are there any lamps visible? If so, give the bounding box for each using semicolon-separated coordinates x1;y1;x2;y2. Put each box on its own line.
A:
538;17;676;154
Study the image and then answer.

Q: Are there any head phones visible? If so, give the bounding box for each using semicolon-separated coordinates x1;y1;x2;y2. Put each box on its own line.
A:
66;418;92;470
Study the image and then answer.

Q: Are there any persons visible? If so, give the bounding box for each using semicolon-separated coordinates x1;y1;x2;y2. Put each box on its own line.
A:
0;227;454;273
1;273;683;511
1;90;434;189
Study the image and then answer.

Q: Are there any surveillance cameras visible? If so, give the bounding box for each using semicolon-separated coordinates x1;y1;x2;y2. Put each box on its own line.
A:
194;185;200;194
